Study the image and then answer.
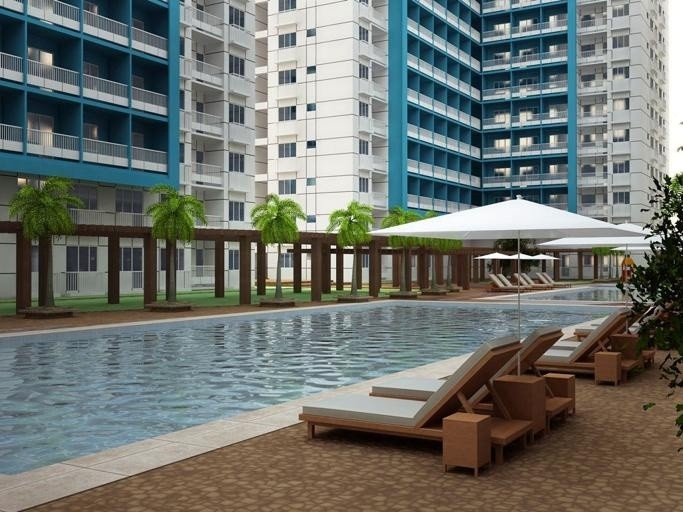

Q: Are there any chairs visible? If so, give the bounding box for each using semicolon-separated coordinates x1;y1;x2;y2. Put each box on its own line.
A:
299;336;533;464
483;269;571;293
368;326;577;434
549;308;655;373
519;309;642;384
